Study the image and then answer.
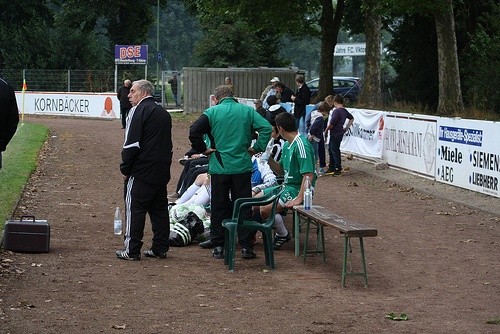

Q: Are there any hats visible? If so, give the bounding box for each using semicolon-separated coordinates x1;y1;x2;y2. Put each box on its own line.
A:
270;76;280;82
254;99;262;104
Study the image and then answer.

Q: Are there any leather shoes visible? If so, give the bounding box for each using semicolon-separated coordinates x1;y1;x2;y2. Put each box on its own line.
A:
241;247;257;258
211;246;225;258
199;239;213;248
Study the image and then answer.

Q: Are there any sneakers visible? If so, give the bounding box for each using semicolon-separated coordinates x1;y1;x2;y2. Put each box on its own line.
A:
116;250;141;261
273;228;291;250
143;250;167;259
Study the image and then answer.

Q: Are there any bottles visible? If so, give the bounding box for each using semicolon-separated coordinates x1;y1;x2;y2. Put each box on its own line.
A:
114;207;122;235
304;176;311;190
304;189;311;210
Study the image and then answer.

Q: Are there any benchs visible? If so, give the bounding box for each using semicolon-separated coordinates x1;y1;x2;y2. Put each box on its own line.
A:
292;204;378;287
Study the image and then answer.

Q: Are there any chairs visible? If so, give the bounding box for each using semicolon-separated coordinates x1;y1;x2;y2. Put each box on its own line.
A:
222;184;286;272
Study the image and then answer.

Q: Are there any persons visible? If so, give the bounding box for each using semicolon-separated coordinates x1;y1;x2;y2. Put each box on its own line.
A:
116;79;173;261
168;73;354;257
117;80;135;129
0;78;19;173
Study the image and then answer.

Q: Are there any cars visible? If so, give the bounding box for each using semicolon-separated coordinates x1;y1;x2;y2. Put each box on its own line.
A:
153;85;166;102
306;76;363;105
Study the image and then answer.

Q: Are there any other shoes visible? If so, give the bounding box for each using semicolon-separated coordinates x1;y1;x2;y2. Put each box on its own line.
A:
121;126;126;129
334;166;344;177
319;167;329;174
324;169;335;175
167;192;179;199
178;158;189;166
